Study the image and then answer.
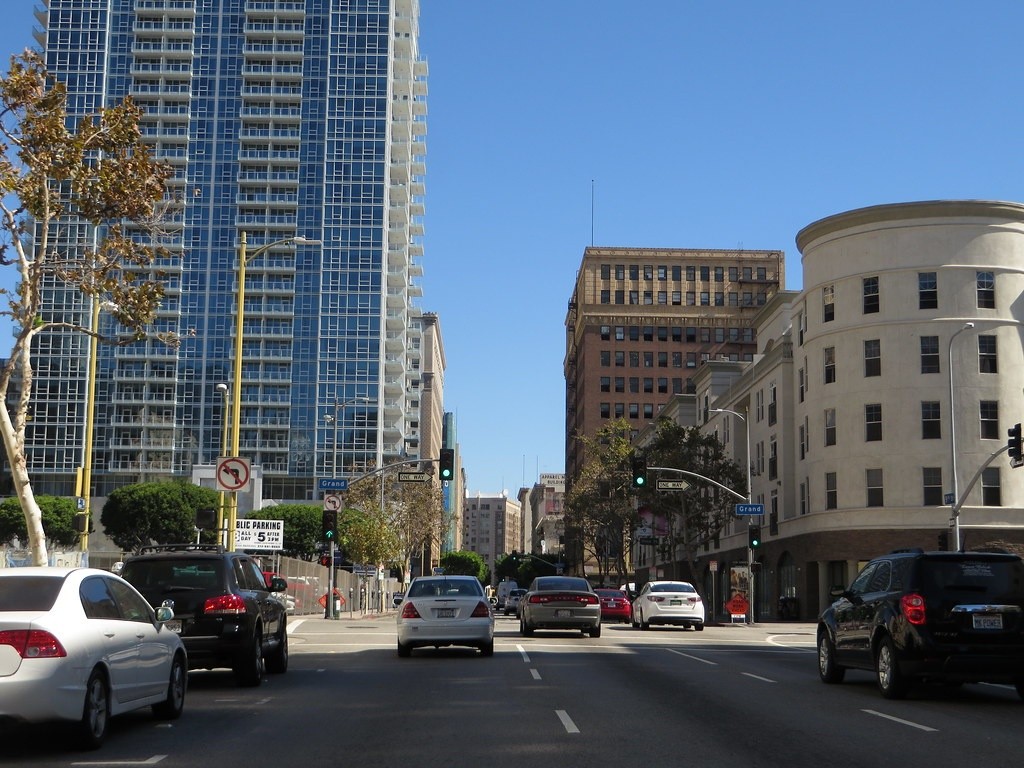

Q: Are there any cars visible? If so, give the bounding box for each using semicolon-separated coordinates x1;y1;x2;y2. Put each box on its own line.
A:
0;567;192;752
592;588;631;625
631;581;704;632
504;576;604;638
390;574;496;658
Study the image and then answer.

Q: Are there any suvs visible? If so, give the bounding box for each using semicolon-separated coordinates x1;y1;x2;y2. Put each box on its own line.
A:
116;544;291;690
816;544;1024;701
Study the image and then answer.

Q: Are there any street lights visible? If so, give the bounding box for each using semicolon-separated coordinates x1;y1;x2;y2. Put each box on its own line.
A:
324;396;378;621
708;408;757;626
948;319;976;552
77;300;121;552
215;383;229;547
226;237;325;551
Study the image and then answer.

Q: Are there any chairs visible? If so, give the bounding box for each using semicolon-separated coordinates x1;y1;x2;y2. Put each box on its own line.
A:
418;585;435;598
457;585;473;595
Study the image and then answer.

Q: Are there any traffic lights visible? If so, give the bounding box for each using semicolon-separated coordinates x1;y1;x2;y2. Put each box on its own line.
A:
749;524;764;549
631;452;647;489
439;448;454;481
1008;423;1022;462
322;510;338;542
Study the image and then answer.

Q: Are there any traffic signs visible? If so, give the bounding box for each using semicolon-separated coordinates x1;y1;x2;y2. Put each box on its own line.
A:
398;472;433;482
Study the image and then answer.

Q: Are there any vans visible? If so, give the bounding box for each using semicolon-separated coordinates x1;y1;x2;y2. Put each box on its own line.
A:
496;582;518;611
